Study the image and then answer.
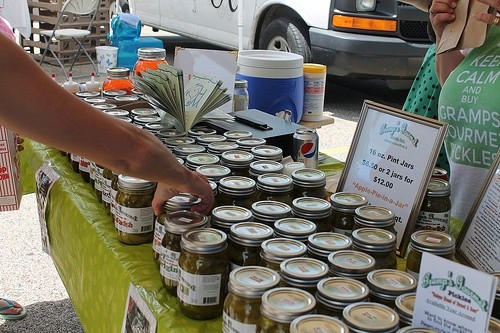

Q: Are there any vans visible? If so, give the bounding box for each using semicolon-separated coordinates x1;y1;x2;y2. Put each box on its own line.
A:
112;0;437;100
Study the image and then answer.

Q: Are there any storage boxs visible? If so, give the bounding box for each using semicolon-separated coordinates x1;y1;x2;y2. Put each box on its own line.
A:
19;0;116;67
110;36;164;72
196;108;316;163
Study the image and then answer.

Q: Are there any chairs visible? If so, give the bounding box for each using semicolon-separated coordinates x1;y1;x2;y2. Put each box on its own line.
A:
40;0;101;80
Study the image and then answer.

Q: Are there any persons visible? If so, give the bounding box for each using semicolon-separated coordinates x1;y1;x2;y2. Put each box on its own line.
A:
0;32;215;320
401;18;474;179
429;0;500;241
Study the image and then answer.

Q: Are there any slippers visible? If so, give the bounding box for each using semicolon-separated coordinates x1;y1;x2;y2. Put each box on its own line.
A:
0;298;27;319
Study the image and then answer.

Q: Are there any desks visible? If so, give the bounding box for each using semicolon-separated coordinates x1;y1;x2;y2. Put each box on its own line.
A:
297;114;336;129
15;137;465;333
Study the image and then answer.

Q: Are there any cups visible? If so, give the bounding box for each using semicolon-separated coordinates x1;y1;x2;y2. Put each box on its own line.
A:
104;47;118;73
95;45;109;73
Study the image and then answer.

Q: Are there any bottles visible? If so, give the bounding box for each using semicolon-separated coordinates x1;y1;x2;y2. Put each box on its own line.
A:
303;64;327;122
82;72;103;97
232;81;249;113
63;72;79;95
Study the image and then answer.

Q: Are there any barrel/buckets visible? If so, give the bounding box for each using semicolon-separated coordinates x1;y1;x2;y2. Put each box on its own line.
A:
236;50;304;124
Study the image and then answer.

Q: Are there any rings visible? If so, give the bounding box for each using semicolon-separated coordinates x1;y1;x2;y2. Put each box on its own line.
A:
494;17;500;25
